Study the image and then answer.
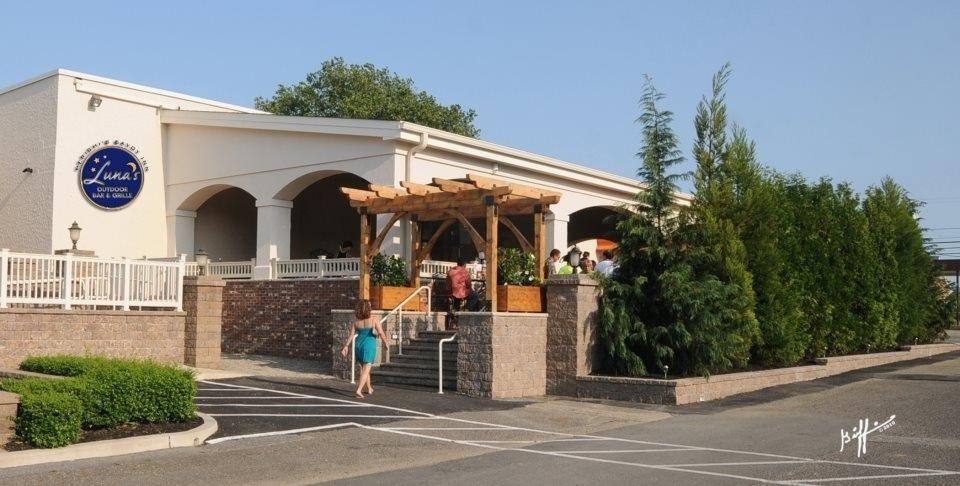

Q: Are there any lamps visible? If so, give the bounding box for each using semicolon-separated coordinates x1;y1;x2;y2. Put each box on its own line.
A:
89;94;102;108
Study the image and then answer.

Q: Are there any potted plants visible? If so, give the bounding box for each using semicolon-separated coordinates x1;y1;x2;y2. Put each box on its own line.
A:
361;250;420;312
486;248;542;312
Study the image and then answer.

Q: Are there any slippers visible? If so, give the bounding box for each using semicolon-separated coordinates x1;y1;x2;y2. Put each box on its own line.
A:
356;383;375;399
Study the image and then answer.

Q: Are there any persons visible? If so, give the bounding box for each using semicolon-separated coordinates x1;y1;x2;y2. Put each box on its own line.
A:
337;240;354;269
340;298;389;398
544;249;621;278
448;257;479;329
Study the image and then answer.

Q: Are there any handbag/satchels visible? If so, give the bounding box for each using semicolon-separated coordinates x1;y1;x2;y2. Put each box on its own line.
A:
371;317;379;337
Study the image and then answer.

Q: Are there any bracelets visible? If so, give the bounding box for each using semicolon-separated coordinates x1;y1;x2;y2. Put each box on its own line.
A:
345;344;350;346
